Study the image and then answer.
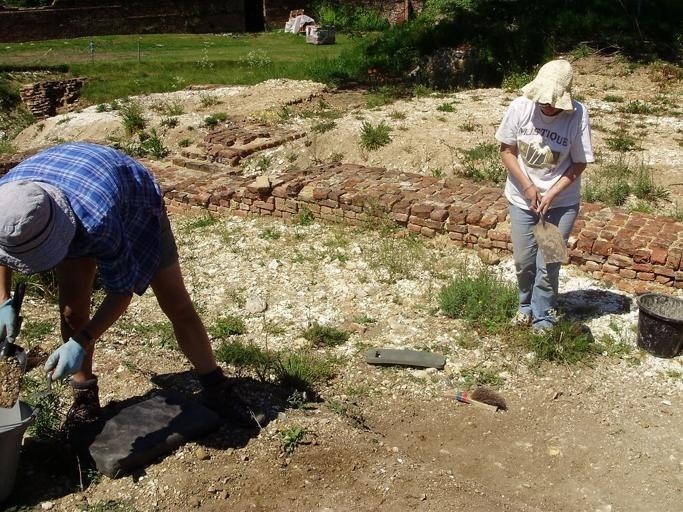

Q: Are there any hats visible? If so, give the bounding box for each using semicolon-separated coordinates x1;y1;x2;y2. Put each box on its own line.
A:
0;181;76;274
520;60;576;112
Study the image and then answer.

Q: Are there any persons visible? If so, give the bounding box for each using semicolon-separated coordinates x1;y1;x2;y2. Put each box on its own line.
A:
0;143;253;433
495;60;596;337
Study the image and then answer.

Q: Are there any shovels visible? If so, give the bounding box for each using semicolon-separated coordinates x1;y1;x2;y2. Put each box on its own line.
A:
531;199;570;265
0;282;28;408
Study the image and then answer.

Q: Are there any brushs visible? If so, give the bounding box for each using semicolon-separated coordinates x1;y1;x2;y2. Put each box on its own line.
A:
444;388;507;412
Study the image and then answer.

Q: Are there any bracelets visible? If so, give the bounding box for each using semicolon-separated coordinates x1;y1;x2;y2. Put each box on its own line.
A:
78;328;96;345
521;182;535;193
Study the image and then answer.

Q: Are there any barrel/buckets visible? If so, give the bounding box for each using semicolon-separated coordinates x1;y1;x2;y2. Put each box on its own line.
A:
638;292;682;358
0;399;43;510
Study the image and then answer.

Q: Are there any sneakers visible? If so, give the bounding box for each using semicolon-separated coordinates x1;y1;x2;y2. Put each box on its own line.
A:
533;329;553;338
508;312;531;327
201;367;252;425
64;375;102;430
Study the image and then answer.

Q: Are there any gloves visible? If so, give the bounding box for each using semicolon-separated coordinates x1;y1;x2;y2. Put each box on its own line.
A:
0;298;23;343
44;337;87;383
